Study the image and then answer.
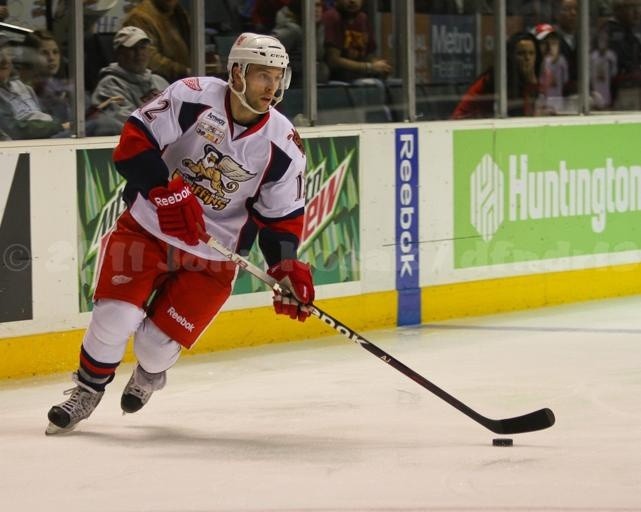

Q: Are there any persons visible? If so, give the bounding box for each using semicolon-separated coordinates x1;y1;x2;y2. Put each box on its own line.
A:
267;0;417;122
446;0;624;120
2;0;201;138
46;32;315;429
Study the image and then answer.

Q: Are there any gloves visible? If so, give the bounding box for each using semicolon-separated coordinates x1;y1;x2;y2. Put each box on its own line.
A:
267;257;315;323
148;176;208;246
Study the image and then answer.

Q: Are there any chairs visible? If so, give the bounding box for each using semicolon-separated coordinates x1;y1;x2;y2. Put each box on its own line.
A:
0;1;475;142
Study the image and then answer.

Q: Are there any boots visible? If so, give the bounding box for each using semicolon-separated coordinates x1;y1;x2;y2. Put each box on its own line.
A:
121;364;166;414
48;383;105;430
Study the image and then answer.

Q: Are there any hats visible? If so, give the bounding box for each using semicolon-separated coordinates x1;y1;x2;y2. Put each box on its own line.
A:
110;25;150;53
530;21;564;40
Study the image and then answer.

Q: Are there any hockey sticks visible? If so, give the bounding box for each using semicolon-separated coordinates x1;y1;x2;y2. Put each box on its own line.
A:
202;234;554;434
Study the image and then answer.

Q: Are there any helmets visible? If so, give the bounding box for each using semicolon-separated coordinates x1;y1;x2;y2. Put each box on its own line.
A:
225;30;294;115
